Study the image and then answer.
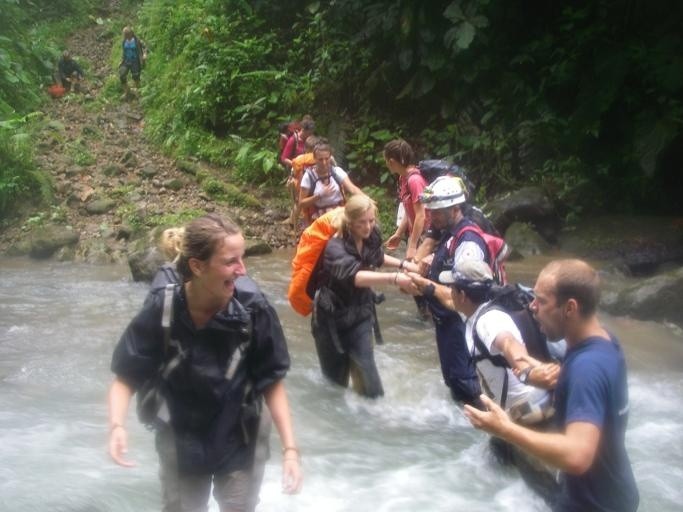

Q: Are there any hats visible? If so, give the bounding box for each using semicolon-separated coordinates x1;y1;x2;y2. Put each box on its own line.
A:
439;259;494;293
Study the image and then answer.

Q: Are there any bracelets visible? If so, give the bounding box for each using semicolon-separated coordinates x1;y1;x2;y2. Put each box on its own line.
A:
111;424;124;428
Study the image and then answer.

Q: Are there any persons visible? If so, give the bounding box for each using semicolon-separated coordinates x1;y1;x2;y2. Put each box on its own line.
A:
282;115;364;218
384;139;502;407
53;50;84;94
106;215;303;512
398;259;640;511
118;26;147;100
308;194;420;397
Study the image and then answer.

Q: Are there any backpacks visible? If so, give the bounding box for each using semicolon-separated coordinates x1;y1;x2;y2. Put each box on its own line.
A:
450;222;510;289
275;121;304;164
475;285;569;409
405;160;475;198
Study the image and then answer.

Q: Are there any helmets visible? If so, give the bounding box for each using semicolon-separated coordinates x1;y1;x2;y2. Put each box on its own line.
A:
418;175;467;211
